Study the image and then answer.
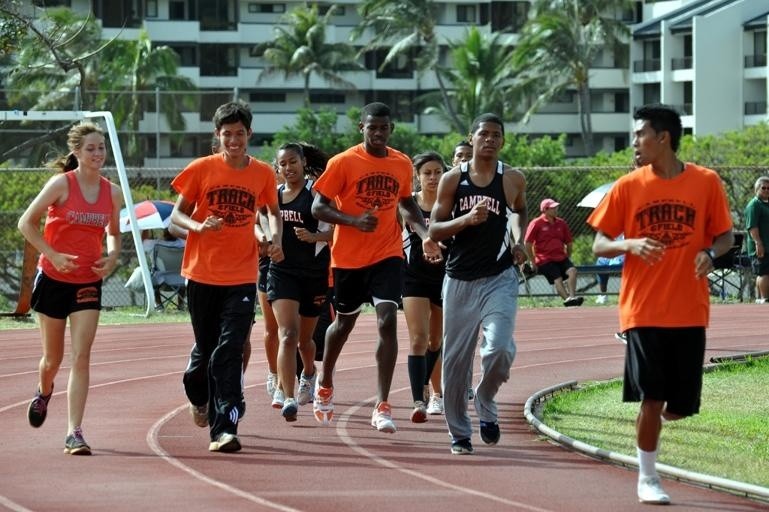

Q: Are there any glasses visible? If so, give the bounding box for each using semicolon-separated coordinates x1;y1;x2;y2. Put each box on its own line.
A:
756;186;769;190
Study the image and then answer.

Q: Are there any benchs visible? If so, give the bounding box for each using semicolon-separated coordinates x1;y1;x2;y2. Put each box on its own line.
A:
519;265;627;307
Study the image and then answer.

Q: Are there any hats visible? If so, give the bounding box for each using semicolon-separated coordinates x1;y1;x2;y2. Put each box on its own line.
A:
539;199;560;212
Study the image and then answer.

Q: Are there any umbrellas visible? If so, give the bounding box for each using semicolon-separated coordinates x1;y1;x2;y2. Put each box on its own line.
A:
576;182;612;208
119;196;176;240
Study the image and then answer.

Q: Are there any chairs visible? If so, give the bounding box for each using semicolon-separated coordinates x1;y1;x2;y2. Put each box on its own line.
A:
710;232;745;302
143;244;189;312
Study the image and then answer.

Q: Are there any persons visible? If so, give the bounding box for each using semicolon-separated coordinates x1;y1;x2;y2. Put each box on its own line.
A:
397;150;455;427
586;103;735;505
524;197;585;307
427;112;529;457
311;101;447;434
140;228;187;314
594;231;624;305
453;141;474;168
253;203;277;398
17;122;124;456
743;176;769;304
168;101;286;453
252;139;336;423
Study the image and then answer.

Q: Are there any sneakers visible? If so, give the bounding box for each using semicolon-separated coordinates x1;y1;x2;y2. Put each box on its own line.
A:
370;402;397;433
282;398;299;423
269;387;285;410
614;333;627;345
467;389;474;401
267;366;280;397
313;372;335;426
26;381;55;429
208;434;242;454
427;394;444;416
564;295;584;307
637;474;672;506
409;401;426;424
480;419;500;445
188;404;209;428
63;426;93;456
297;365;317;406
449;435;475;456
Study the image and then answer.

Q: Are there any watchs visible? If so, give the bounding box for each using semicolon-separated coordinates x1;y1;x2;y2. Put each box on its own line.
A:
702;247;716;260
